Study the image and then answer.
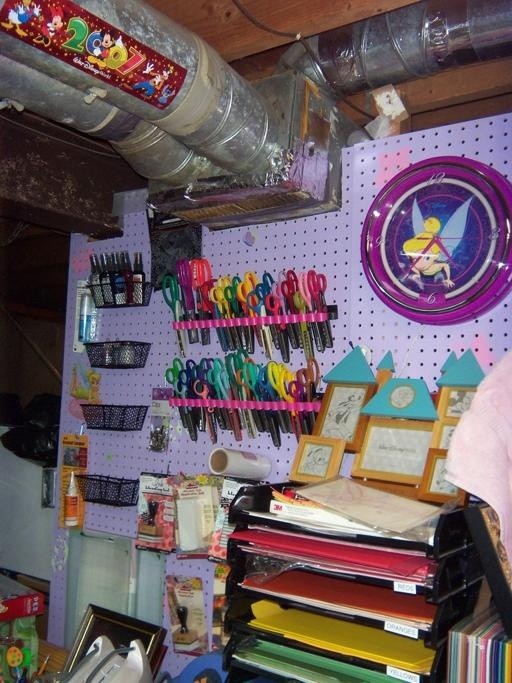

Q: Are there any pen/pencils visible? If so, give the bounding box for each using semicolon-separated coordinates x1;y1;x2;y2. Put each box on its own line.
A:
208;556;227;563
37;655;50;675
284;490;307;501
135;545;171;556
16;667;27;683
178;554;210;560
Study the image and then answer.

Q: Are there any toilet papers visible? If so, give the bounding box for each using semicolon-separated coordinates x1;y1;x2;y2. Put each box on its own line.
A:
207;448;272;482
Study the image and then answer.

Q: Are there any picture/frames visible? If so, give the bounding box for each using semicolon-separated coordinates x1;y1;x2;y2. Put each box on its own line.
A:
58;603;168;683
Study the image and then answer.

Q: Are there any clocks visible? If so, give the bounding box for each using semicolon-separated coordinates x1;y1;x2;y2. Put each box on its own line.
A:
360;156;512;327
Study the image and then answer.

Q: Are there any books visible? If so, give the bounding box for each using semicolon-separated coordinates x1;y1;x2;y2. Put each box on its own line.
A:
268;498;376;533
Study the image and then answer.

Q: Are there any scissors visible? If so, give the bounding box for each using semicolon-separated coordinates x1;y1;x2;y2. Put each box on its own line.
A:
165;349;324;447
162;258;333;364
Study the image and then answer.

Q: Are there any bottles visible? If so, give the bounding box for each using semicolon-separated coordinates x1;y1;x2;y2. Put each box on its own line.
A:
89;249;144;310
64;471;79;526
99;335;138;365
75;293;94;342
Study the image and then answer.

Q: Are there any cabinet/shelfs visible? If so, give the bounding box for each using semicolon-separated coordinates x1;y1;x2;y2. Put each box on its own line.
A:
220;480;490;682
